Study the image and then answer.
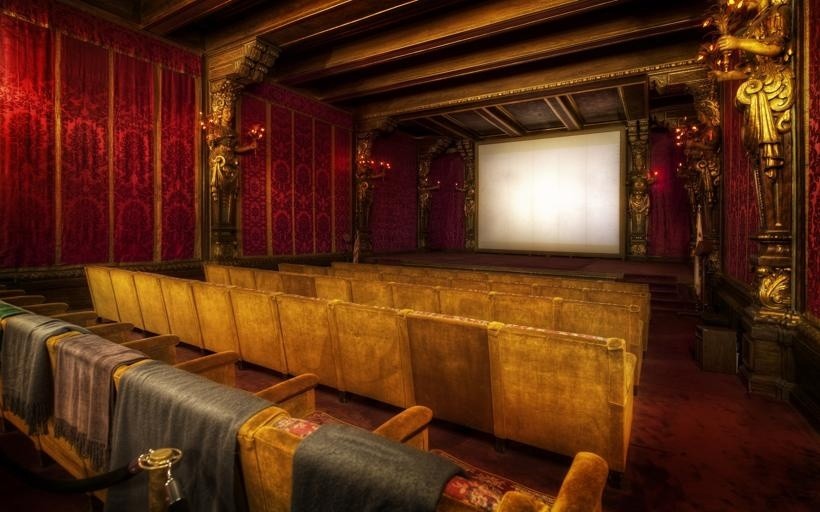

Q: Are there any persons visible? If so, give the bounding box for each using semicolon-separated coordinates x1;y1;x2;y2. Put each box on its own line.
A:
417;165;441;230
355;140;389;229
676;129;699;211
625;150;658;243
680;102;721;210
205;94;259;229
455;166;476;235
707;0;796;182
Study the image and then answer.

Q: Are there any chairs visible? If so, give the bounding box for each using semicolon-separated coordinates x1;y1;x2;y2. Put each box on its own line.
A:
87;263;652;475
3;278;607;511
